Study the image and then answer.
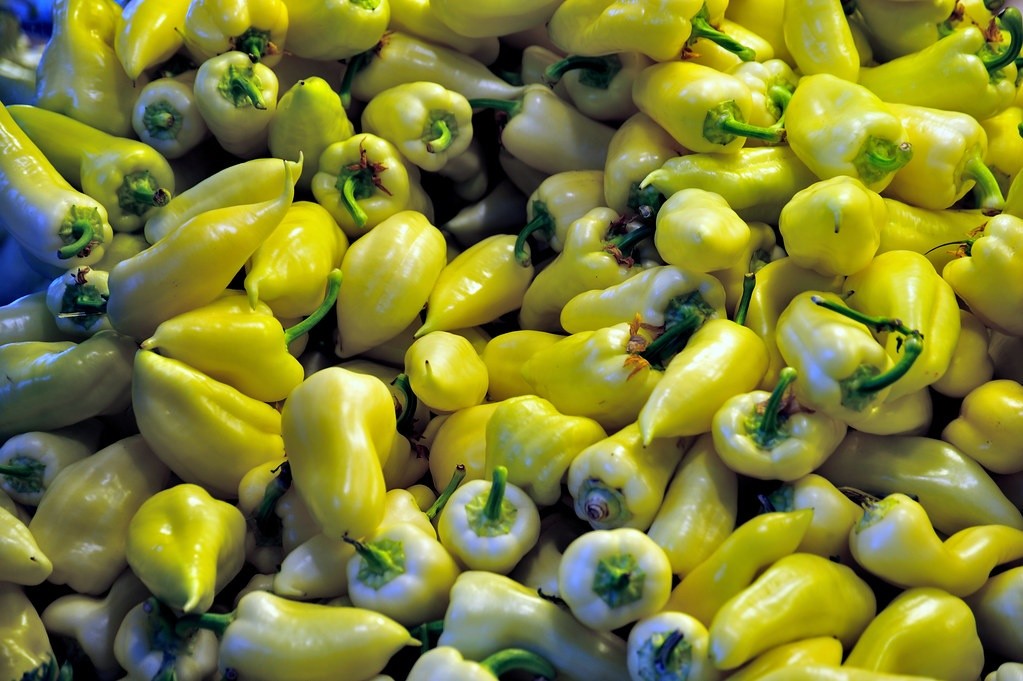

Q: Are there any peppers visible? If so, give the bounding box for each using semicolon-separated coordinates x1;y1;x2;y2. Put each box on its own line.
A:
0;0;1023;681
